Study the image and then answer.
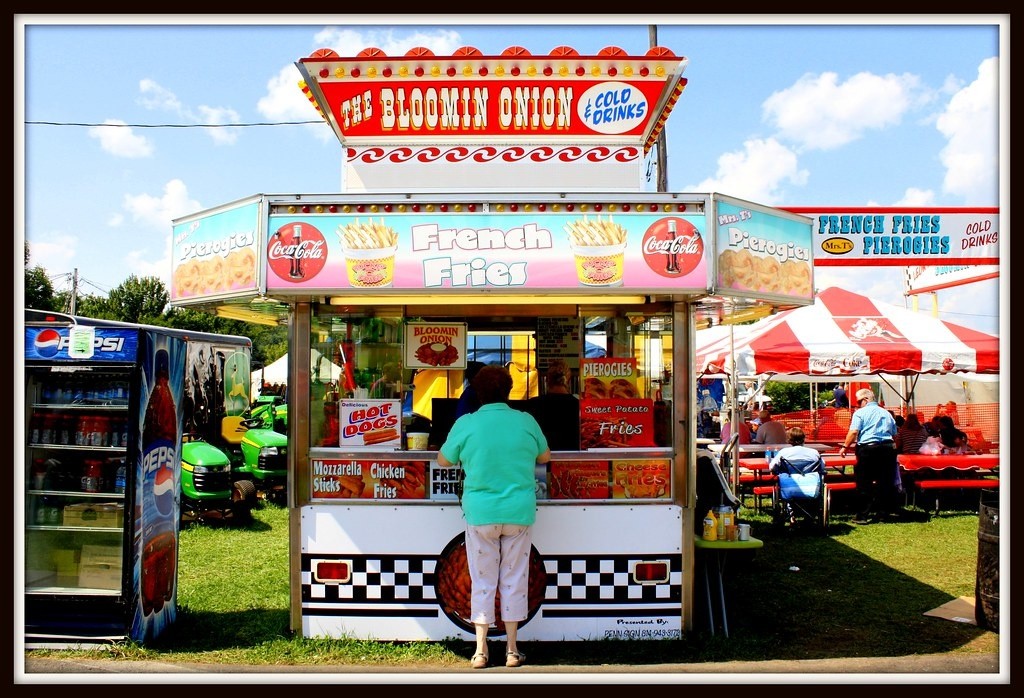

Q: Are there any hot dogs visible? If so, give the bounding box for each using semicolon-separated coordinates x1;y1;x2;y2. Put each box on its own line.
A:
363;428;400;446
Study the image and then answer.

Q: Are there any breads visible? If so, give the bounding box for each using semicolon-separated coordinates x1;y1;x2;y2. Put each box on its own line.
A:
719;249;812;297
175;247;255;296
584;377;635;400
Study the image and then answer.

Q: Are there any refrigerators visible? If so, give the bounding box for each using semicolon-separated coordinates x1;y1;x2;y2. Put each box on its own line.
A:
25;321;188;653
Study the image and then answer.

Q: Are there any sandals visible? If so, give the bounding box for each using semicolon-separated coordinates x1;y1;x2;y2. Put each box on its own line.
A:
471;652;488;668
506;651;526;666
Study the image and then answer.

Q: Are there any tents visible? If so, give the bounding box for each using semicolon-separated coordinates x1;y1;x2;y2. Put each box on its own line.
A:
251;349;341;399
696;286;1000;485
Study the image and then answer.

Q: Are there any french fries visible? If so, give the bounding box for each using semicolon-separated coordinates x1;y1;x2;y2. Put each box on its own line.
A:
337;475;365;498
336;218;399;249
563;214;628;247
380;461;424;493
495;552;545;629
437;543;471;619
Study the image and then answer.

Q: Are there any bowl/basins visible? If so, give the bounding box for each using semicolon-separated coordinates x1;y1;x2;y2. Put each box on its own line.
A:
406;432;430;450
570;241;625;286
343;244;397;288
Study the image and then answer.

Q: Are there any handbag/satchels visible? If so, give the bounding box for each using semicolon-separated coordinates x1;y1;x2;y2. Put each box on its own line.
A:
918;436;941;455
778;471;821;499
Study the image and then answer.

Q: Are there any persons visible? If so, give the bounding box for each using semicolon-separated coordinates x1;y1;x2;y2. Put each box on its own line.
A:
888;409;967;455
532;359;580;451
437;366;550;668
769;427;825;522
456;361;487;420
745;382;754;411
829;382;847;409
753;410;786;458
369;362;400;398
936;401;959;425
839;389;898;524
720;410;751;459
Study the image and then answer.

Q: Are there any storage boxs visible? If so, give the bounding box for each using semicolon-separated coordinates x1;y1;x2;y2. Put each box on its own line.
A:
63;506;123;529
80;539;123;567
78;563;121;590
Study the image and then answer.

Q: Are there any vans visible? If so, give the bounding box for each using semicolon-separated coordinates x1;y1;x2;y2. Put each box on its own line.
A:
722;380;776;415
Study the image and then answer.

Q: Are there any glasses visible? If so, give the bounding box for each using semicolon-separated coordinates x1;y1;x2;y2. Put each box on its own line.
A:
858;398;865;404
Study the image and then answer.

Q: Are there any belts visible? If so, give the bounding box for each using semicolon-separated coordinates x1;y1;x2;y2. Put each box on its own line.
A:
867;443;892;448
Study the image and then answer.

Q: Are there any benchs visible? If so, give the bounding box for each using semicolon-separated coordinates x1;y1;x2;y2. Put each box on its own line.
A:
730;473;1000;518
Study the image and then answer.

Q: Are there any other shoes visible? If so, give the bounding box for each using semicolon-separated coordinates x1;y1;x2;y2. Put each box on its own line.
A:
851;514;867;524
879;517;884;523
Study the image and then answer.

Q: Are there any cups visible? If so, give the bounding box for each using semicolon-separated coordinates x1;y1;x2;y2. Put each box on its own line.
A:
724;525;741;540
739;524;750;541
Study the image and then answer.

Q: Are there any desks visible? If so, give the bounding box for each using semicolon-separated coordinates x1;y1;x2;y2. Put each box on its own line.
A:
838;442;856;450
697;438;715;447
695;534;764;644
897;453;1000;508
708;443;833;455
733;456;858;517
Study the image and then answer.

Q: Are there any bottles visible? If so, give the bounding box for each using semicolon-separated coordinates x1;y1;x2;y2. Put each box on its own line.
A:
30;372;134;525
290;225;305;278
773;447;779;456
665;219;682;273
765;447;771;463
141;349;177;617
703;510;717;540
698;389;719;428
